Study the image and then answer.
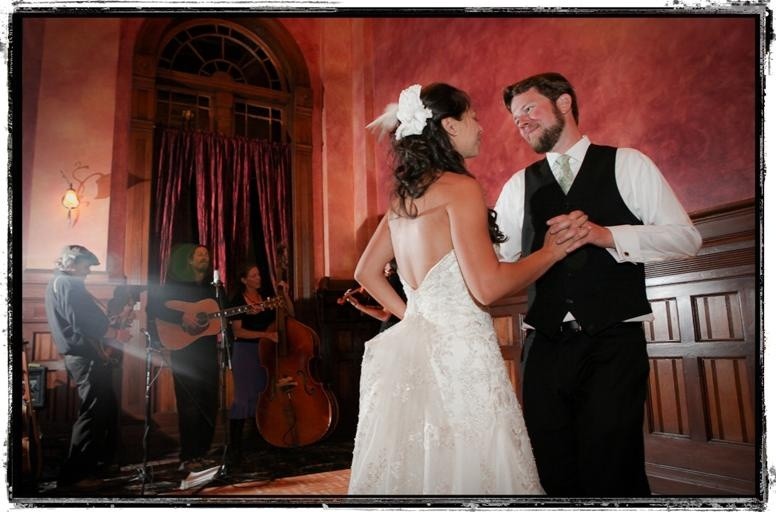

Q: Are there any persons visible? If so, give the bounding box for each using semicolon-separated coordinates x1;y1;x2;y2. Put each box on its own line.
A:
347;257;407;333
346;83;595;498
484;71;702;497
142;243;229;474
227;261;297;464
44;243;140;484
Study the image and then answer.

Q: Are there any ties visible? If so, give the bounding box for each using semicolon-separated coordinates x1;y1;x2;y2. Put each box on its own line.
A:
553;154;573;194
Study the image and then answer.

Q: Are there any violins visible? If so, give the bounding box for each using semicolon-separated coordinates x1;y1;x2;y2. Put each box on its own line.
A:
337;278;391;310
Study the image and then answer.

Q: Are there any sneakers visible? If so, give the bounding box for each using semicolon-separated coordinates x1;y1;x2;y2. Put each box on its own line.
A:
74;478;104;489
178;458;216;472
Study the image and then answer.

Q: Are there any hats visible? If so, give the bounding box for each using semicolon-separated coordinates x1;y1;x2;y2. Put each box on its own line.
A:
65;244;100;265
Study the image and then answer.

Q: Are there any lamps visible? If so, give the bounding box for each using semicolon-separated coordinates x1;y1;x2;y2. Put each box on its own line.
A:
62;182;79;225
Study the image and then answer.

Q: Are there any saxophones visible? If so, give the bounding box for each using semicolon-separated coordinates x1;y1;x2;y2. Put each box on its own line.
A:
101;295;137;363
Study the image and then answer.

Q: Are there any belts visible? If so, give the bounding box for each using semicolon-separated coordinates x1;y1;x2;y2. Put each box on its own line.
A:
559;320;582;335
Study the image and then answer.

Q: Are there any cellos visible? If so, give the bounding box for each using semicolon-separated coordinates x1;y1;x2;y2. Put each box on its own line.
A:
255;240;341;449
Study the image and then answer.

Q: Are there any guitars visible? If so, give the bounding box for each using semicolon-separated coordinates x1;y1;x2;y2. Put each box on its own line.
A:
152;294;288;352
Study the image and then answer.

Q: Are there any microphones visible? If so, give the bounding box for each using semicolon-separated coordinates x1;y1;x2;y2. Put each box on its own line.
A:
214;269;219;299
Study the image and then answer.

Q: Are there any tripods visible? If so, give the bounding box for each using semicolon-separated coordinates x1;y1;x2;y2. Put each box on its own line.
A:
189;299;274;496
119;330;175;497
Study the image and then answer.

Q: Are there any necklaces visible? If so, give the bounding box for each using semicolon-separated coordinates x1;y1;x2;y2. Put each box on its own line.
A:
246;293;262;304
